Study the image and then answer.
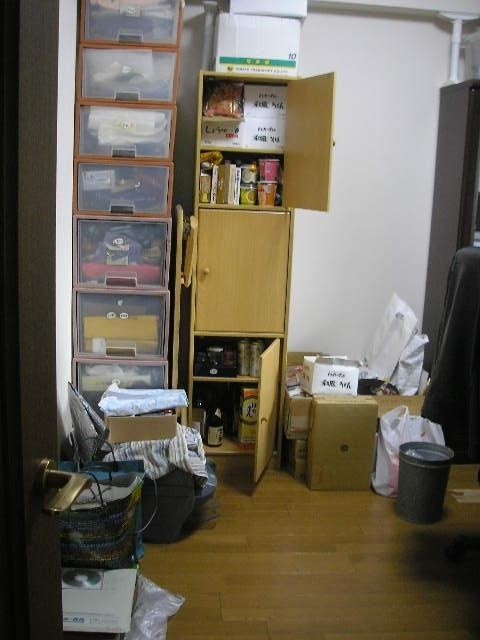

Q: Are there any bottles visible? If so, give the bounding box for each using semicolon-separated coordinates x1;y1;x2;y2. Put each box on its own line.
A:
206;397;225;446
192;399;206;443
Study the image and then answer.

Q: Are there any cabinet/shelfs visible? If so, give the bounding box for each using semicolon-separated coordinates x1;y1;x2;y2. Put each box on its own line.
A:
187;70;334;481
421;79;480;376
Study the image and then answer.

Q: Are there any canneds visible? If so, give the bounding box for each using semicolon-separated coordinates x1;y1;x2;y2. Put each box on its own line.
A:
237;340;265;378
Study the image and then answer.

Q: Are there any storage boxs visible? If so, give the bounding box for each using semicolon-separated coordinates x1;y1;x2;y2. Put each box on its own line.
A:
283;351;425;491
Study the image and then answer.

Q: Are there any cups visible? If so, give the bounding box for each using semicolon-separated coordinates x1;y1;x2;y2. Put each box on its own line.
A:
256;181;278;207
257;156;280;181
241;160;257;185
239;183;256;204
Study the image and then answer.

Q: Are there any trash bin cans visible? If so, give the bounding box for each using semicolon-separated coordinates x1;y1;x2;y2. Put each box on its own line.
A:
395;442;455;525
